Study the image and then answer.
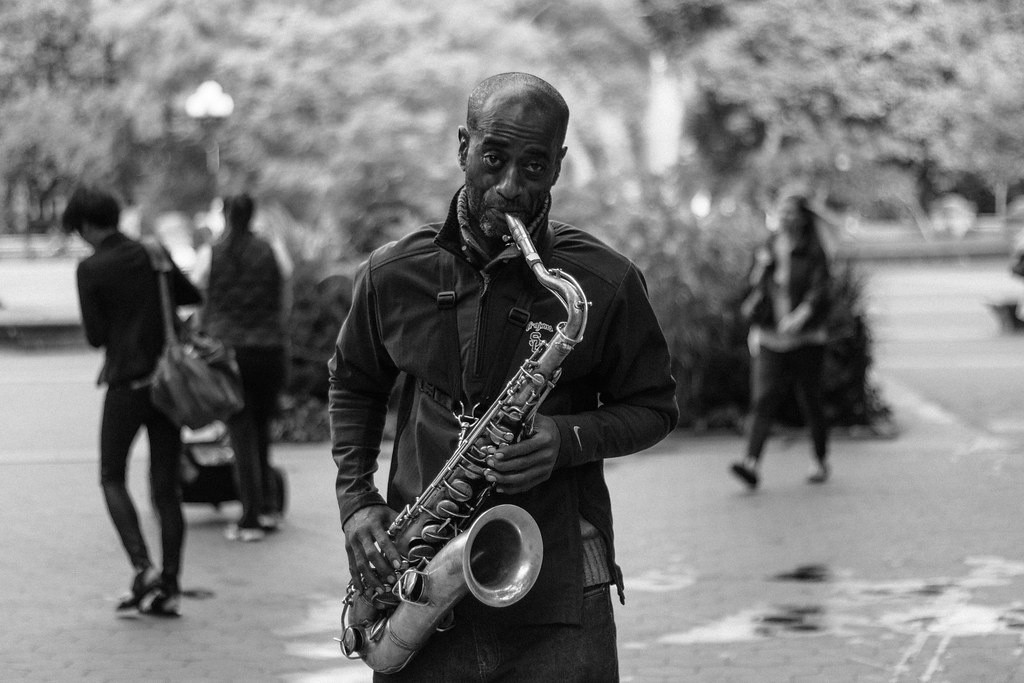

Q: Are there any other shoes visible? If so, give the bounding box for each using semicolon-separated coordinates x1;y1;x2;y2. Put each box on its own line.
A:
142;590;183;617
224;513;276;541
732;461;760;488
114;565;161;612
810;467;830;482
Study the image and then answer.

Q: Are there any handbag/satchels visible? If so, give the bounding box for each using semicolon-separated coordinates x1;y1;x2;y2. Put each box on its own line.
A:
151;343;245;431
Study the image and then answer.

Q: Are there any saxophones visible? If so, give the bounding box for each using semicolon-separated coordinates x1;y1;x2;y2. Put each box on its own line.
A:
333;203;602;678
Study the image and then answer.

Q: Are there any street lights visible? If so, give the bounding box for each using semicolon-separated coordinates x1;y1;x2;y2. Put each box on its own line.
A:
184;80;234;175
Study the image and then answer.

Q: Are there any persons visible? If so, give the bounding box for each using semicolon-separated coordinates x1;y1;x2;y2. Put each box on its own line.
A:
192;192;292;542
63;185;205;615
728;196;831;488
327;72;679;683
